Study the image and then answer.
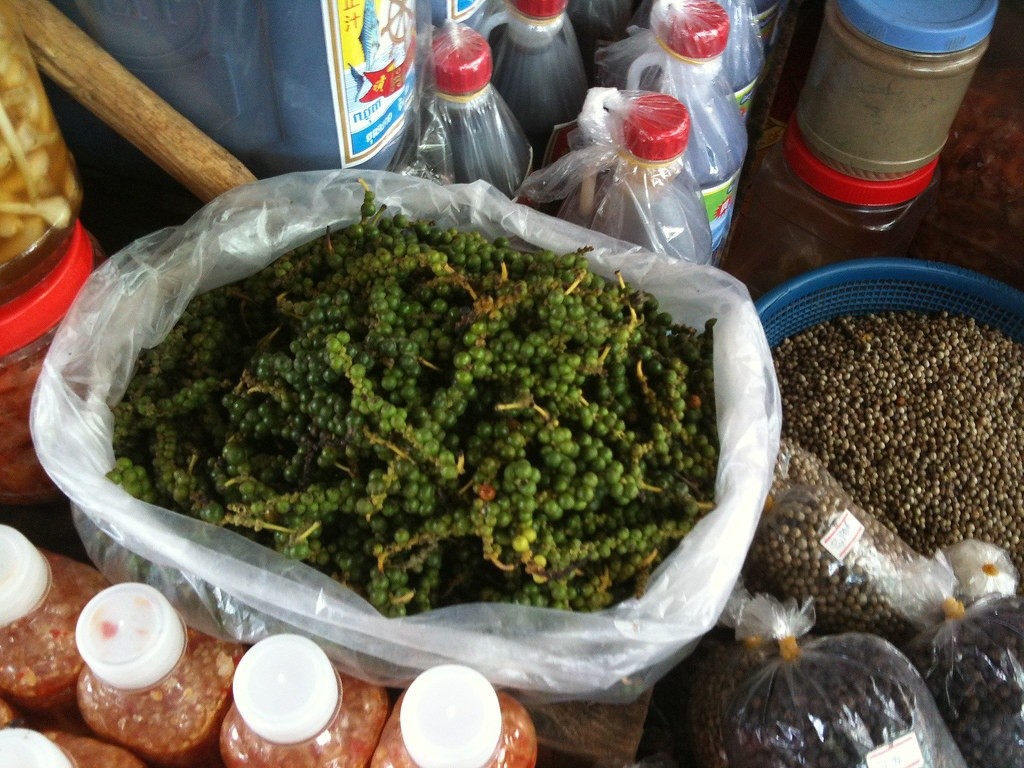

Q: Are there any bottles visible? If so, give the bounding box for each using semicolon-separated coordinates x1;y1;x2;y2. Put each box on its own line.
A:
796;0;998;182
0;1;804;767
